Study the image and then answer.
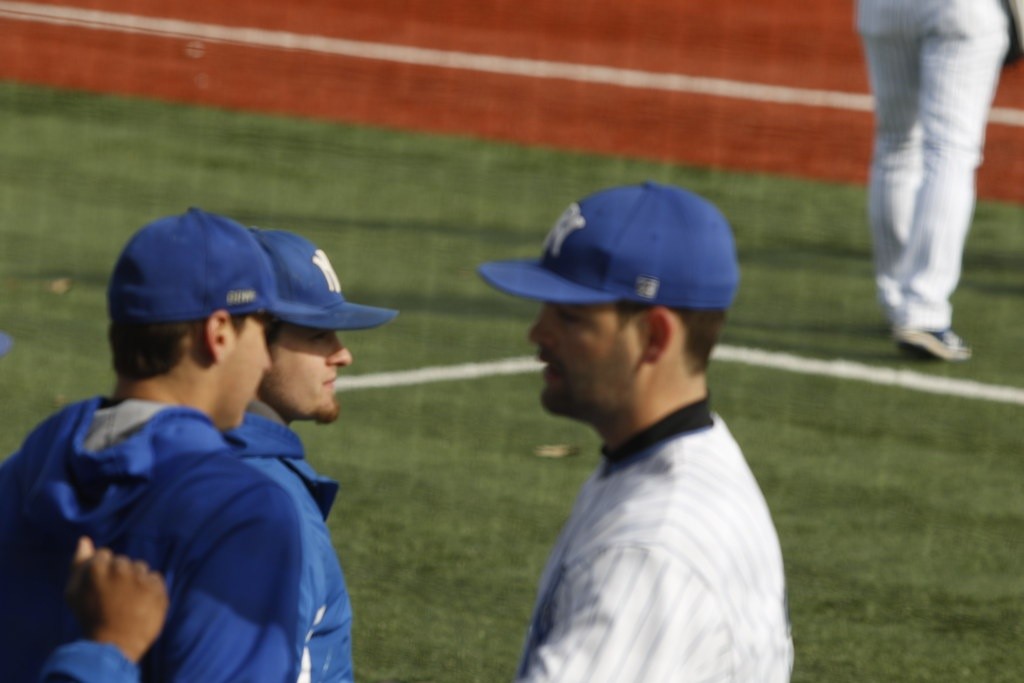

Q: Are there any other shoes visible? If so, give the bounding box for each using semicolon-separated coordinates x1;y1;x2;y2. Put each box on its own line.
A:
896;327;974;360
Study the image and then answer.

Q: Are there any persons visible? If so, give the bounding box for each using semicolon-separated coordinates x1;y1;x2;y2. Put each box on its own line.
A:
0;331;169;682
0;207;326;683
226;225;397;682
476;180;796;683
853;0;1024;362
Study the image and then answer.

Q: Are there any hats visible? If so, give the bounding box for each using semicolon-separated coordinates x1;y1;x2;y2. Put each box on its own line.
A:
104;204;324;322
480;181;738;309
248;229;399;330
